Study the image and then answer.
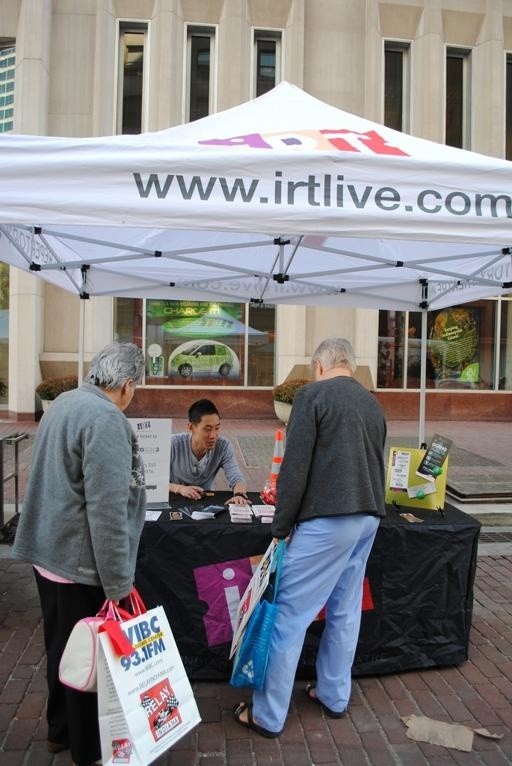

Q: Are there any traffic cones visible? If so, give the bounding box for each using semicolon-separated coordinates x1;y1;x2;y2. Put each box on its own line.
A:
269;430;286;487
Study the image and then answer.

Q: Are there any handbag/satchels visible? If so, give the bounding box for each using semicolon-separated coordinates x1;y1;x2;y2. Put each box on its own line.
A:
229;540;287;692
58;599;112;692
95;591;202;766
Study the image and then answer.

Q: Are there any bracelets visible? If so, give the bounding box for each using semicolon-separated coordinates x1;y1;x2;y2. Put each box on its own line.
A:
234;491;249;500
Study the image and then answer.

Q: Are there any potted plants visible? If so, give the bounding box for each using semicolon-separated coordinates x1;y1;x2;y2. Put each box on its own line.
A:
35;376;78;413
272;378;311;428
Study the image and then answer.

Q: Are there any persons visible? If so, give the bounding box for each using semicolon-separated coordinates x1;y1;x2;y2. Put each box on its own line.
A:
167;398;249;507
9;341;150;765
230;335;388;741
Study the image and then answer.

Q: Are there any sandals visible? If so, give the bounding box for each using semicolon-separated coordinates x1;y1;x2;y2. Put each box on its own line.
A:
306;683;346;718
233;702;282;738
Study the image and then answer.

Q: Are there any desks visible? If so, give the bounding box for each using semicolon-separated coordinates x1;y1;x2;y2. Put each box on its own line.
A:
135;489;482;681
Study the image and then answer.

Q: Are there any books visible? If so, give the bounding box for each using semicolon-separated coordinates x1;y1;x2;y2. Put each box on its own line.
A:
177;504;276;524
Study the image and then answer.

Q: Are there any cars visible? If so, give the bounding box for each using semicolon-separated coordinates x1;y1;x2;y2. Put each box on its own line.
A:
172;343;234;377
